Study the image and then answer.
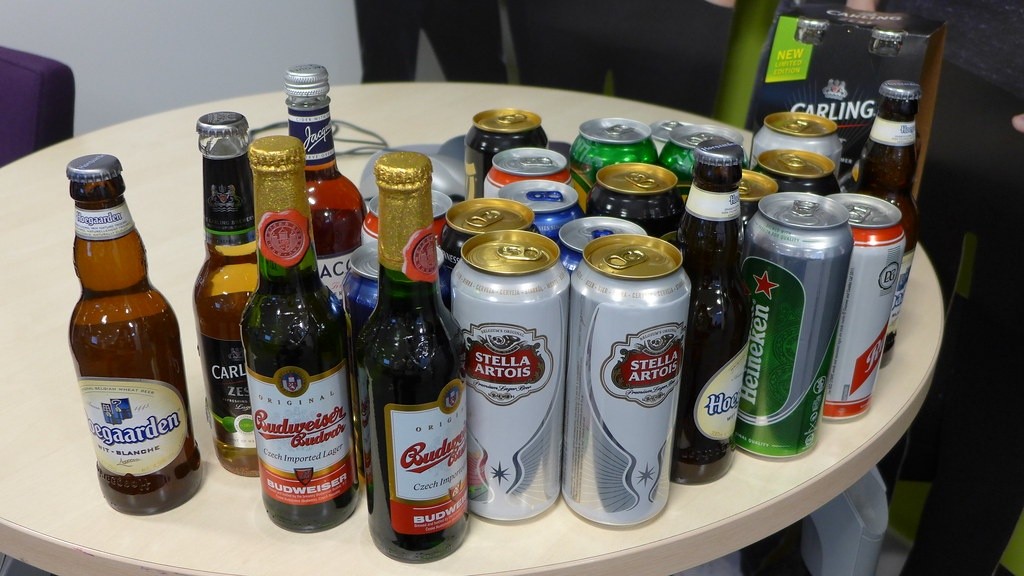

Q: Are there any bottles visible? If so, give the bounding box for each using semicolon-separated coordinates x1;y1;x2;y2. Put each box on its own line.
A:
66;153;202;519
668;138;751;486
359;151;471;564
191;110;259;476
247;136;361;535
284;63;365;302
837;81;922;369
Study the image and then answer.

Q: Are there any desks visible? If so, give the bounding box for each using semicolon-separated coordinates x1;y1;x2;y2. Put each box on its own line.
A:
0;79;946;576
